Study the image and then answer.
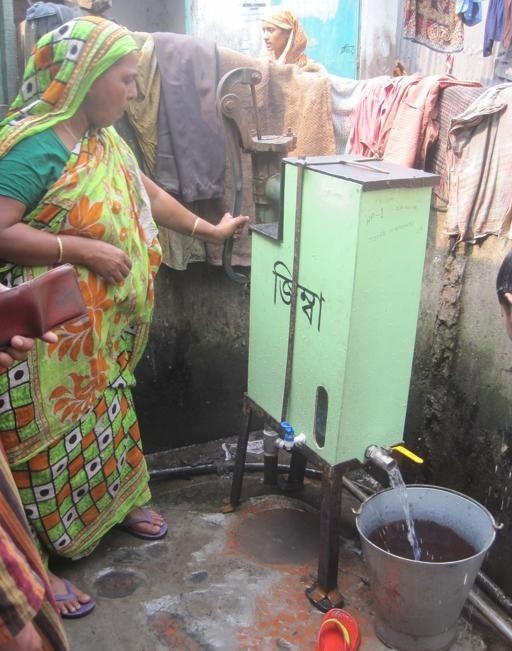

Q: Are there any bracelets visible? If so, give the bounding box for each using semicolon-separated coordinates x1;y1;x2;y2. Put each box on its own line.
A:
189;217;201;238
53;233;65;266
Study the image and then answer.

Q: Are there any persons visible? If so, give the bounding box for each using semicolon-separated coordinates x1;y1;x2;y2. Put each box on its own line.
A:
0;15;252;623
258;6;318;69
495;251;511;308
1;281;70;651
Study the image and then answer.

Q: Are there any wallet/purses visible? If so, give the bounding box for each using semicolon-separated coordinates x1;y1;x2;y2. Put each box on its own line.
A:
0;262;89;357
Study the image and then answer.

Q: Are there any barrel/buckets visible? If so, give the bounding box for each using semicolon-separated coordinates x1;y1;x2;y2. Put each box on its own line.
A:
351;483;503;651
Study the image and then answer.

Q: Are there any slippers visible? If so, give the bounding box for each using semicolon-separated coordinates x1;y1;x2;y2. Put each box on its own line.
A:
48;577;96;619
317;607;361;651
119;507;169;541
